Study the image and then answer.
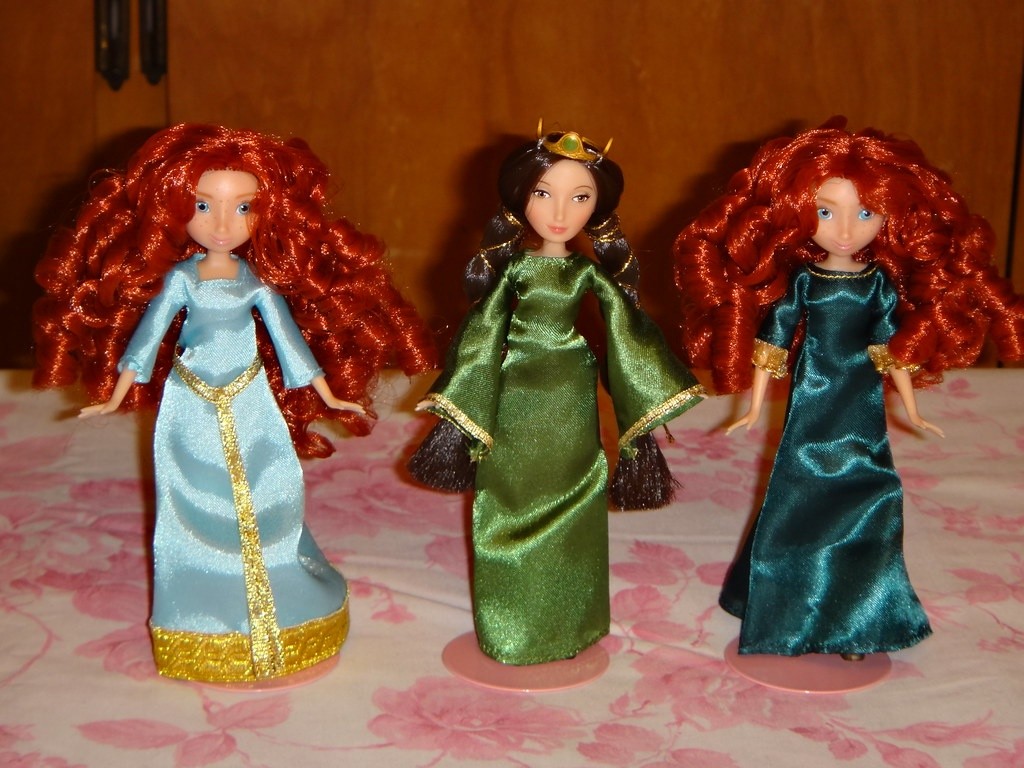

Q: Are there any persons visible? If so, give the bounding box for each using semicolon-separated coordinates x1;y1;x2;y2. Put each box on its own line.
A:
405;117;704;665
35;125;438;690
671;122;1024;664
